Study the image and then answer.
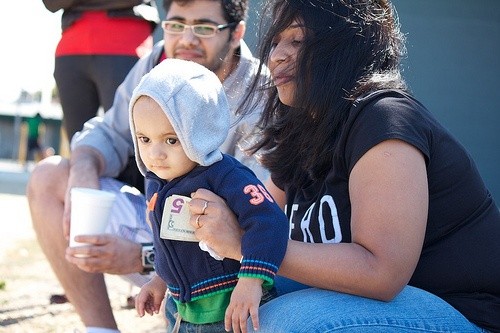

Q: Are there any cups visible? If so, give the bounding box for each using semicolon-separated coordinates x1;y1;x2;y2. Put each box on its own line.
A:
69;187;115;257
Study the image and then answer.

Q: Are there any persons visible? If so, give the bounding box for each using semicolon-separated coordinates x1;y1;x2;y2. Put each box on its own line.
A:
40;1;160;309
27;0;276;333
189;0;500;332
128;57;290;333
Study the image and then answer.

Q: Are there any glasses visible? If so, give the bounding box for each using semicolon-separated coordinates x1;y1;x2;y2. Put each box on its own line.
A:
161;20;238;38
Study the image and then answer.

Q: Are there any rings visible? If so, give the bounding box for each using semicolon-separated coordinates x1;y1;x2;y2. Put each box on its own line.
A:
202;202;208;212
196;215;200;226
